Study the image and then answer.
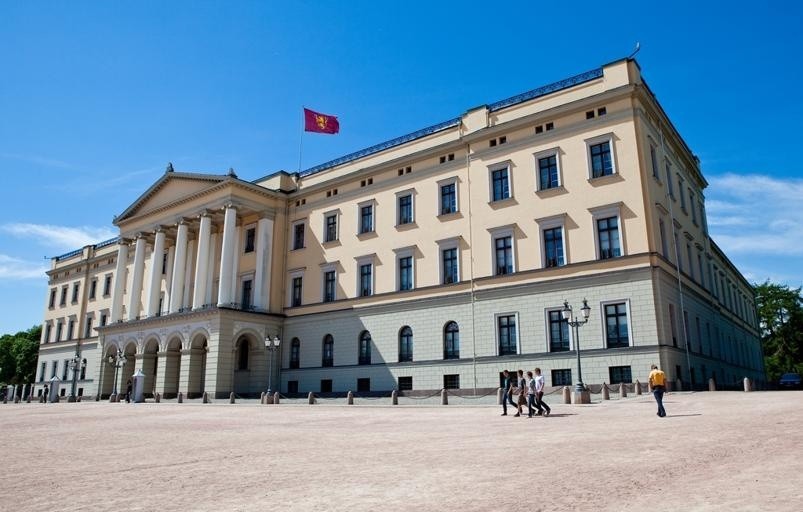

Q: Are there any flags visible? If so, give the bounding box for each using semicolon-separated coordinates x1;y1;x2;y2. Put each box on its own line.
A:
303;108;339;135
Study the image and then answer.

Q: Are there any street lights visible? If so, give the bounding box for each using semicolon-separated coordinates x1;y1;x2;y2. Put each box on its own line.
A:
561;299;590;391
108;350;126;394
264;334;280;395
67;354;86;396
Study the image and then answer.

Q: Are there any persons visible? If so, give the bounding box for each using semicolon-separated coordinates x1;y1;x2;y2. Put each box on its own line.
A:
512;369;536;417
500;369;522;416
42;383;48;403
125;379;132;403
522;371;545;418
648;364;668;416
533;367;551;416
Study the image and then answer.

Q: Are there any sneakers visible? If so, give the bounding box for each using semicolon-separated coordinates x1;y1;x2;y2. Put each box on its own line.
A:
501;407;551;417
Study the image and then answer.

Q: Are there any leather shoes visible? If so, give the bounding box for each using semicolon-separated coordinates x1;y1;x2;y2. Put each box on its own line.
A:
656;412;666;417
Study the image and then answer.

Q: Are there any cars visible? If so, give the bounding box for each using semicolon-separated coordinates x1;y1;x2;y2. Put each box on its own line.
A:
779;373;800;388
